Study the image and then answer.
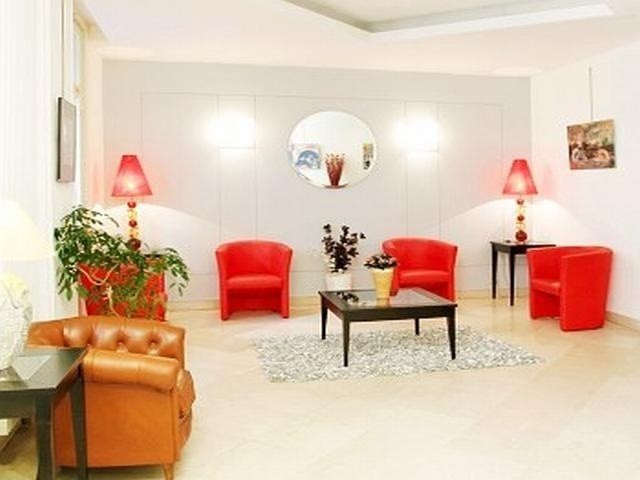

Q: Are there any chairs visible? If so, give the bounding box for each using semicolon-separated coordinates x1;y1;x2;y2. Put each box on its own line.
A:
77;255;168;326
23;314;198;480
382;237;458;301
524;244;614;332
214;237;295;321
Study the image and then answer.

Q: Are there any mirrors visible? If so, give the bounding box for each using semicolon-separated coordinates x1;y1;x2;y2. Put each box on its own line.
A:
286;108;379;193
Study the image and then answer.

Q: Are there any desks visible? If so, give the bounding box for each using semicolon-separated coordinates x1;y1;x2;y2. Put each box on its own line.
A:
0;345;92;480
316;287;459;367
488;241;558;308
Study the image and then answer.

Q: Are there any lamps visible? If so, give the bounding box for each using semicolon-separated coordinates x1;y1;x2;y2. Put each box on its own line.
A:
111;153;154;243
0;200;58;371
501;156;540;243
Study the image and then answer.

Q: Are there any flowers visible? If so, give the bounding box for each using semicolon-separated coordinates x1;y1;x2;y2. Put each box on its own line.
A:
315;223;367;273
364;252;400;271
324;151;346;159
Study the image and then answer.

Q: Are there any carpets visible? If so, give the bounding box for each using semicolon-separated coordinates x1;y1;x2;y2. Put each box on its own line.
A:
248;324;542;384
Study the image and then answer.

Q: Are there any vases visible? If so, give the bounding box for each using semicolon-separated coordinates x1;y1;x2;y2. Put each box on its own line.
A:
324;271;353;291
371;268;394;300
323;156;345;186
